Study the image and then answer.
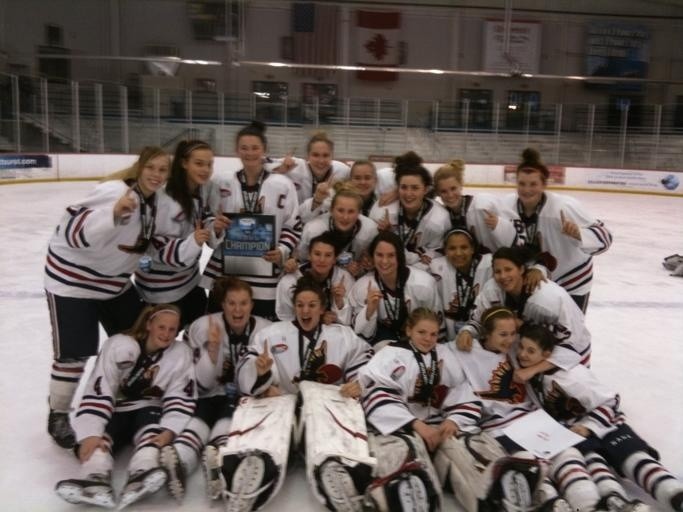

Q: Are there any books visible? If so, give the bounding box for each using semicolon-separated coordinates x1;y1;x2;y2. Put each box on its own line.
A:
221;212;276;278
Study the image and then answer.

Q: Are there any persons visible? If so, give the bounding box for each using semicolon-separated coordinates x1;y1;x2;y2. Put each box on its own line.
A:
429;227;557;341
441;308;600;512
434;156;527;252
202;120;301;319
518;324;683;512
299;159;379;229
159;280;274;502
455;246;591;382
349;232;448;353
500;148;613;317
303;190;380;277
44;146;173;449
55;303;197;509
135;140;214;334
276;231;354;325
357;307;532;512
226;277;375;512
264;131;348;202
369;151;452;265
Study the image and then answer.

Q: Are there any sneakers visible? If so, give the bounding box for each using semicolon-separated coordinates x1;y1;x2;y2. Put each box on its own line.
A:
119;466;169;504
158;444;185;498
47;410;76;449
55;480;113;504
502;472;532;511
224;456;266;511
393;474;428;511
202;443;223;500
321;461;361;511
549;499;573;511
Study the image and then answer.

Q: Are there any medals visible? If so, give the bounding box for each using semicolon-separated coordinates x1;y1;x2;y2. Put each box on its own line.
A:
227;323;250;394
137;187;157;270
